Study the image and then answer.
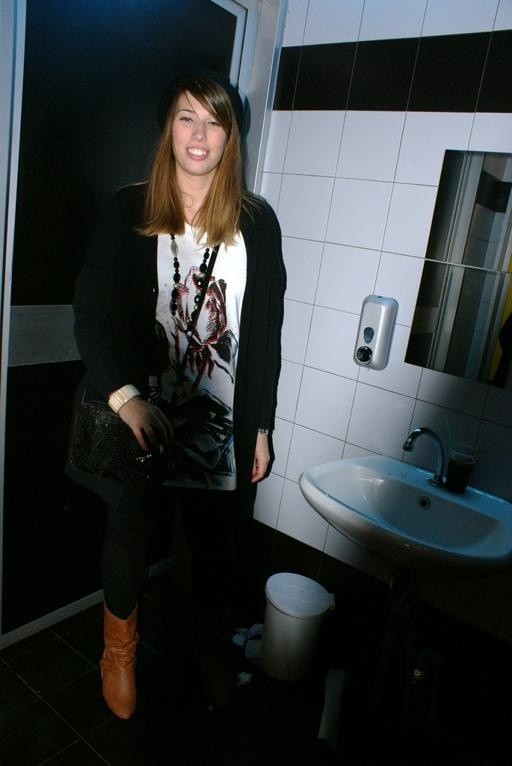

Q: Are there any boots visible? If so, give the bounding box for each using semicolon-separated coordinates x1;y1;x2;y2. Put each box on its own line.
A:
100;598;138;718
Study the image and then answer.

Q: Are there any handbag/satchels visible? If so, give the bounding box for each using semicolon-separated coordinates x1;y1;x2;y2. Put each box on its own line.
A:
69;400;184;489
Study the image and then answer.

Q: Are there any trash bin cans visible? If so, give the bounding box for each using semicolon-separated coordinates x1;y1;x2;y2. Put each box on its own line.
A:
262;573;336;682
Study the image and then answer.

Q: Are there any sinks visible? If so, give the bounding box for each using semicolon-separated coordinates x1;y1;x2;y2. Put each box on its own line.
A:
299;456;512;580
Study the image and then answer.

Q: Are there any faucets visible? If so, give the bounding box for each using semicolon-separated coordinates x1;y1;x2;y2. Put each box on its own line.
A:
403;426;444;485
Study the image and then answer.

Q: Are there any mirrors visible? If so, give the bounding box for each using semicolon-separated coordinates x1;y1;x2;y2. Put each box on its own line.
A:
404;150;511;390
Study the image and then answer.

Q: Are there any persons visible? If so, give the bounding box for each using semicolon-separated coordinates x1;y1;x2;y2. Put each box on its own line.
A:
64;74;288;721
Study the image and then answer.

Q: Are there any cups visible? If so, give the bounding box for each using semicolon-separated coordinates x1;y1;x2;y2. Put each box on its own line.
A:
444;444;480;496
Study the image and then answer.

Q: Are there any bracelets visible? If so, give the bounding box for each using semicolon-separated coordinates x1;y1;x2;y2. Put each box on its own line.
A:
258;428;269;435
